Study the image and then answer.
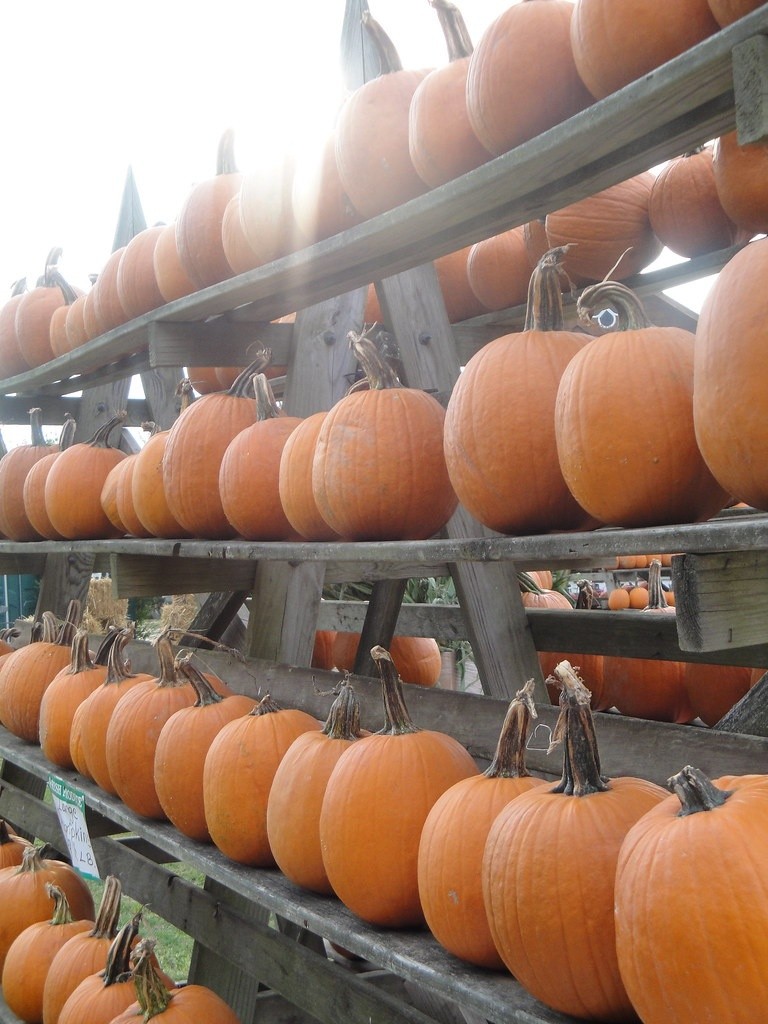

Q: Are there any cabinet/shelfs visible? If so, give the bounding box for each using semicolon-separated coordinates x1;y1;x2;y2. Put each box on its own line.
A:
0;1;768;1024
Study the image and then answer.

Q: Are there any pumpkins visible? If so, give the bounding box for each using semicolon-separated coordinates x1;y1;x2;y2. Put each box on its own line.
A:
0;0;768;1024
